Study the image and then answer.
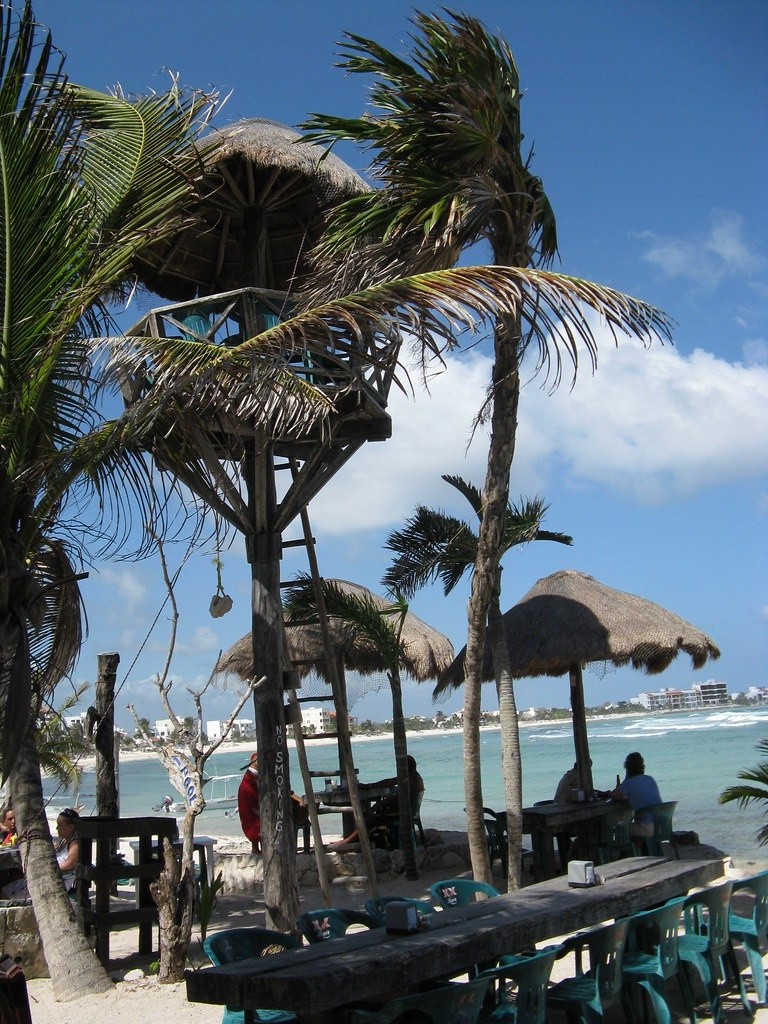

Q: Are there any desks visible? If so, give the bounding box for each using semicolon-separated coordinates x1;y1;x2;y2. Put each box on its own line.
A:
498;791;627;878
183;848;723;1022
0;838;67;907
130;836;220;910
303;781;398;850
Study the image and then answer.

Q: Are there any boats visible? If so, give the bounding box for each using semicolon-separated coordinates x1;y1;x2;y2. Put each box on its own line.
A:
153;769;246;813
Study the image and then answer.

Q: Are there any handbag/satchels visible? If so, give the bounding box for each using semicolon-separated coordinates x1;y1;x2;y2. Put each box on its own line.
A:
670;830;700;846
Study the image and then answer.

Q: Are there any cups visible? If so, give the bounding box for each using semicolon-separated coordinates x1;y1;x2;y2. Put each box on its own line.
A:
331;779;337;789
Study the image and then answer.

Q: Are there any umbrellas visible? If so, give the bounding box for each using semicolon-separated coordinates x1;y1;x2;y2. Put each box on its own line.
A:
433;568;722;802
209;580;455;846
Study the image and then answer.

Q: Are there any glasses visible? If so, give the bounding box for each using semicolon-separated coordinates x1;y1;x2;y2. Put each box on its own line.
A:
64;808;72;816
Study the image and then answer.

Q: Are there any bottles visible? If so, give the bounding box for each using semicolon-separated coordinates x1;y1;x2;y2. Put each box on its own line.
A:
616;775;622;788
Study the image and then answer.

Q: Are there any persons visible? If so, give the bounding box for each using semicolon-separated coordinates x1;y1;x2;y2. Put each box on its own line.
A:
318;755;426;850
0;806;15;844
0;808;82;899
238;752;312;843
554;756;611;863
611;751;664;855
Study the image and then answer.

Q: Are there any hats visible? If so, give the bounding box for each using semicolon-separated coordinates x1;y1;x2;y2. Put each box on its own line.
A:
251;753;258;760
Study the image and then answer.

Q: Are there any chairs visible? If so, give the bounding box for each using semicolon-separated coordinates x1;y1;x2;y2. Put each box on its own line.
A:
190;787;768;1022
